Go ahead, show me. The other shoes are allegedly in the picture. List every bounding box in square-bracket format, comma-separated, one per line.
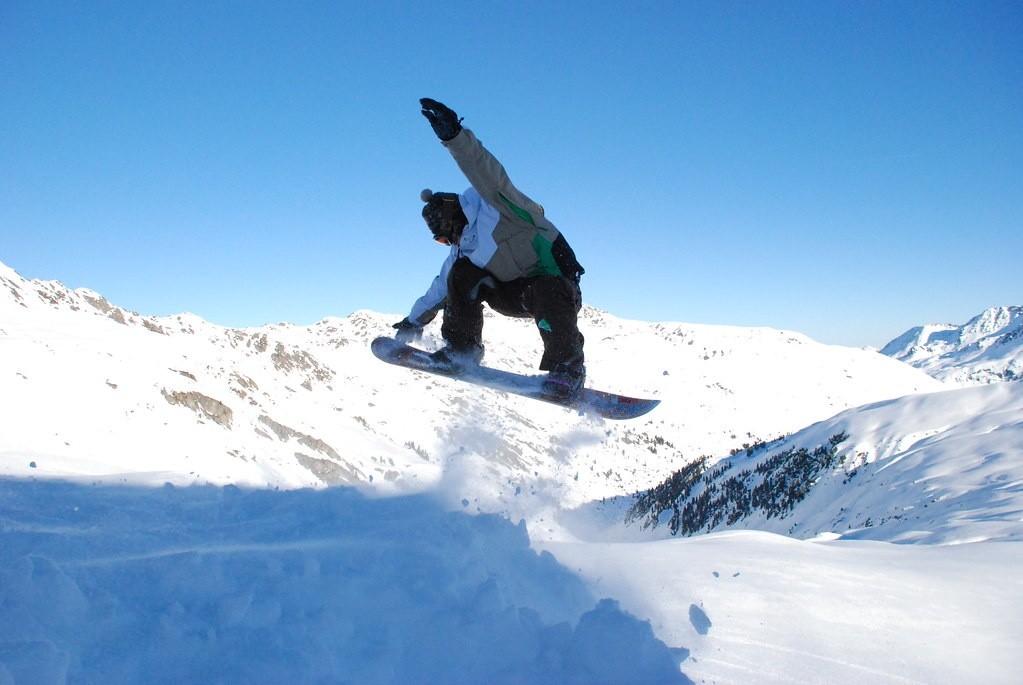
[541, 368, 586, 400]
[431, 337, 484, 373]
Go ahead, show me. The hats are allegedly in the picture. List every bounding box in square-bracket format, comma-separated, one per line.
[420, 188, 460, 237]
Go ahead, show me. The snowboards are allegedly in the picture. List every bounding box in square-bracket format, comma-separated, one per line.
[371, 336, 661, 420]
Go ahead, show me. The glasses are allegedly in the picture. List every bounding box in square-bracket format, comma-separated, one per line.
[432, 234, 454, 247]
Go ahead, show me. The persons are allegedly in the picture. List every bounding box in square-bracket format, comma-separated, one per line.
[391, 97, 585, 403]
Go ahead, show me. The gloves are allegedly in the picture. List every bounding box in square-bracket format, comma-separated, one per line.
[419, 97, 465, 141]
[392, 316, 423, 345]
